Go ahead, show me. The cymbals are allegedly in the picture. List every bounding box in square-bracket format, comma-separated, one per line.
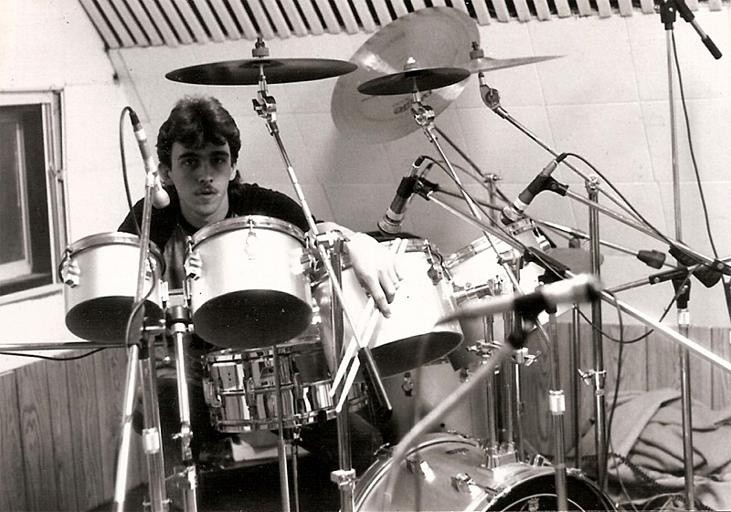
[330, 6, 481, 145]
[465, 52, 564, 75]
[359, 68, 472, 94]
[164, 56, 355, 85]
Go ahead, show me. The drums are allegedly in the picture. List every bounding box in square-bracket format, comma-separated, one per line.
[306, 238, 462, 383]
[441, 216, 584, 371]
[343, 425, 619, 512]
[199, 336, 371, 433]
[183, 214, 312, 350]
[58, 230, 166, 344]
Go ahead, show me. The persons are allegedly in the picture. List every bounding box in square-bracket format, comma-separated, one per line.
[117, 95, 404, 481]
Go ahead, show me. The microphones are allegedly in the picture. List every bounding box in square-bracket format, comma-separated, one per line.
[376, 162, 422, 238]
[405, 169, 430, 210]
[134, 122, 172, 210]
[500, 159, 559, 226]
